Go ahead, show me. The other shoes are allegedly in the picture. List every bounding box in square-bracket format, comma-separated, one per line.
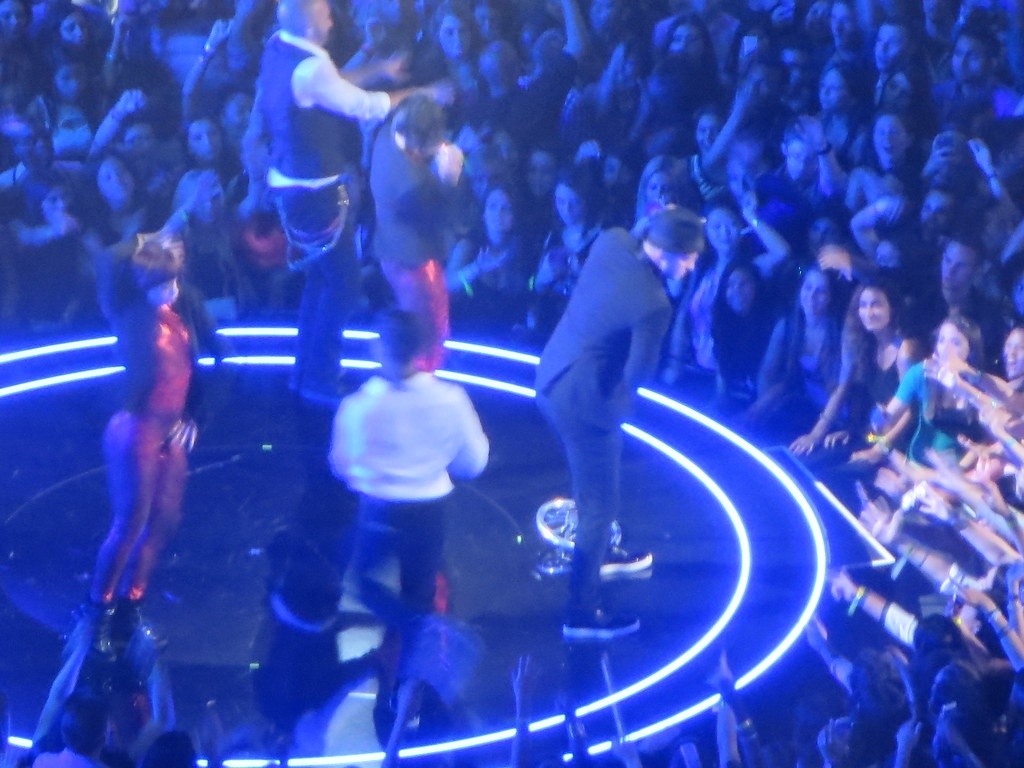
[600, 544, 654, 574]
[289, 368, 350, 411]
[564, 612, 640, 637]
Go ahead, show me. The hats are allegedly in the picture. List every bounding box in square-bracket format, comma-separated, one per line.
[648, 206, 705, 254]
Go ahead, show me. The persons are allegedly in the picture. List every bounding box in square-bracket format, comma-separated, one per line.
[91, 228, 227, 651]
[535, 209, 704, 636]
[367, 97, 449, 367]
[255, 1, 408, 407]
[272, 307, 492, 665]
[0, 0, 1024, 768]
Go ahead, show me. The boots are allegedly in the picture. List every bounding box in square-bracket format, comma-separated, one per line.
[75, 596, 119, 667]
[117, 596, 169, 651]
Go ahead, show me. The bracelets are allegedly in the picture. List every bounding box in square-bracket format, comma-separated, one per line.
[988, 174, 995, 179]
[977, 371, 982, 386]
[820, 414, 833, 423]
[868, 432, 891, 451]
[753, 219, 758, 225]
[977, 392, 981, 400]
